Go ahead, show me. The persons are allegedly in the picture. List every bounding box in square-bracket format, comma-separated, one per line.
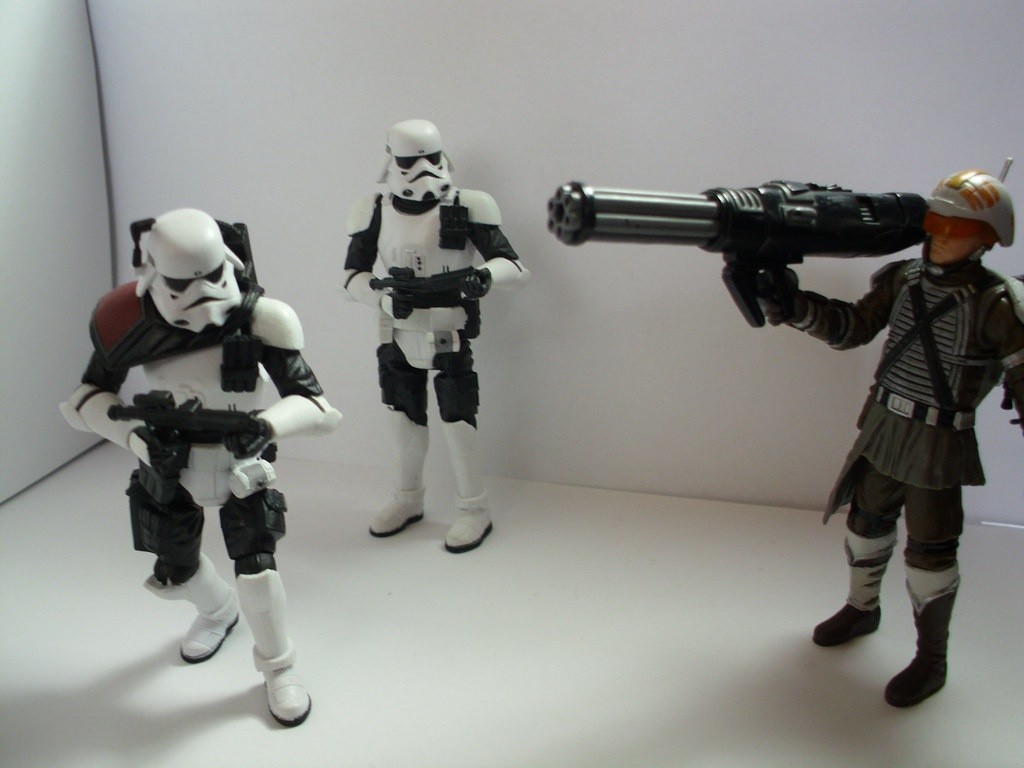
[343, 118, 531, 553]
[69, 209, 342, 727]
[757, 168, 1024, 709]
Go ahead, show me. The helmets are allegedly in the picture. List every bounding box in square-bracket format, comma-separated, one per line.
[377, 119, 455, 201]
[926, 169, 1014, 247]
[136, 208, 245, 333]
